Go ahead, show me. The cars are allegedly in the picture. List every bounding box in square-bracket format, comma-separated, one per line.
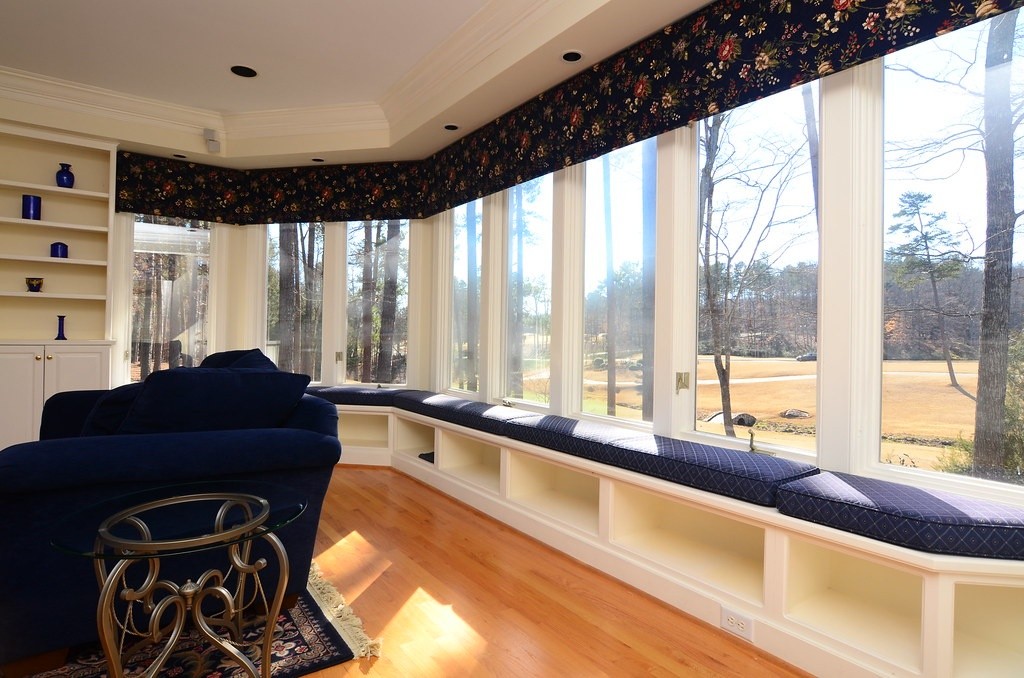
[796, 353, 817, 362]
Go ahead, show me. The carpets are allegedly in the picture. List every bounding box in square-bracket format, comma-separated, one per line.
[30, 561, 379, 678]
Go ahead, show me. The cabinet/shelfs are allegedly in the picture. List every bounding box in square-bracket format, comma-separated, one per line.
[0, 124, 119, 454]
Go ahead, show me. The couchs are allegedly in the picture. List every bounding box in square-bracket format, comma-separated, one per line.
[0, 395, 341, 668]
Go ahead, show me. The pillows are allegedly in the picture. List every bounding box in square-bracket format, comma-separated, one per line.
[77, 349, 310, 437]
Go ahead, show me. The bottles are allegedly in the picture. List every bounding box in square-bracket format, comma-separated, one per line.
[25, 278, 43, 292]
[54, 315, 67, 340]
[55, 162, 74, 188]
[50, 242, 68, 258]
[21, 195, 42, 220]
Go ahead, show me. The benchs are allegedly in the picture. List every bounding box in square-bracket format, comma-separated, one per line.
[303, 383, 1024, 678]
